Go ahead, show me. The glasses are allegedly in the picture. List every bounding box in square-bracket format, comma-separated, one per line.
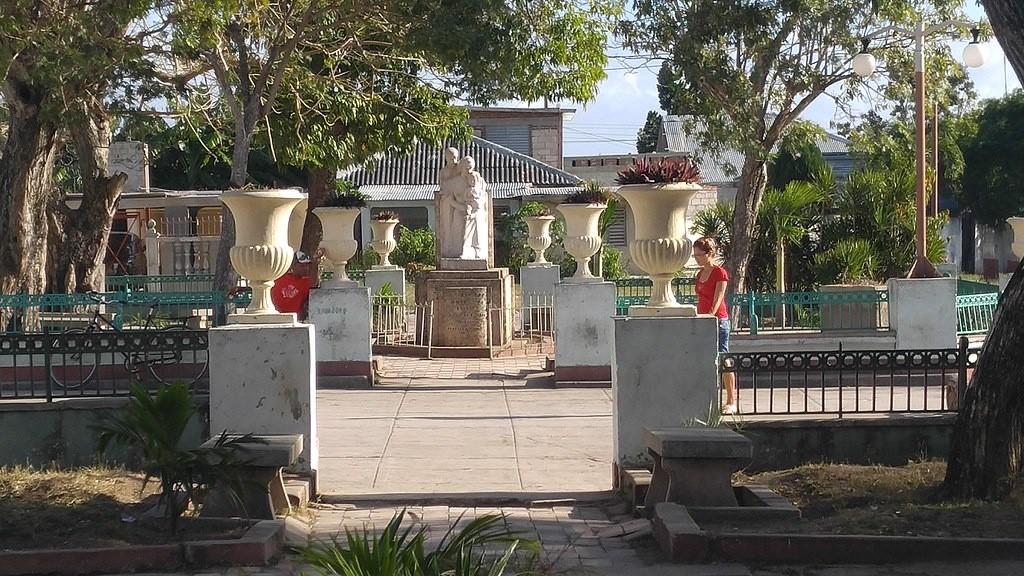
[692, 251, 709, 258]
[292, 255, 310, 266]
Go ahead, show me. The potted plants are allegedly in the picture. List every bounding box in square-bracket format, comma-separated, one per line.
[522, 209, 556, 266]
[311, 188, 367, 289]
[218, 184, 305, 324]
[368, 210, 400, 270]
[613, 154, 703, 317]
[556, 179, 612, 282]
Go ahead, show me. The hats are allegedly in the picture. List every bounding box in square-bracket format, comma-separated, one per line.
[293, 250, 312, 265]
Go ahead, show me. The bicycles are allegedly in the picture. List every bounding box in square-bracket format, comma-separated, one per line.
[47, 290, 210, 389]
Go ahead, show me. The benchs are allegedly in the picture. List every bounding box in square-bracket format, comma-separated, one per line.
[645, 425, 753, 514]
[199, 433, 304, 520]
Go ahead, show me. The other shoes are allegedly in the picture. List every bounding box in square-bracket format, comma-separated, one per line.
[723, 404, 736, 413]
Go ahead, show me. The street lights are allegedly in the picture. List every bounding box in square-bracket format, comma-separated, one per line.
[851, 11, 987, 278]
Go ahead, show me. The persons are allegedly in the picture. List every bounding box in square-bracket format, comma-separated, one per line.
[693, 237, 736, 413]
[270, 249, 323, 320]
[440, 148, 487, 258]
